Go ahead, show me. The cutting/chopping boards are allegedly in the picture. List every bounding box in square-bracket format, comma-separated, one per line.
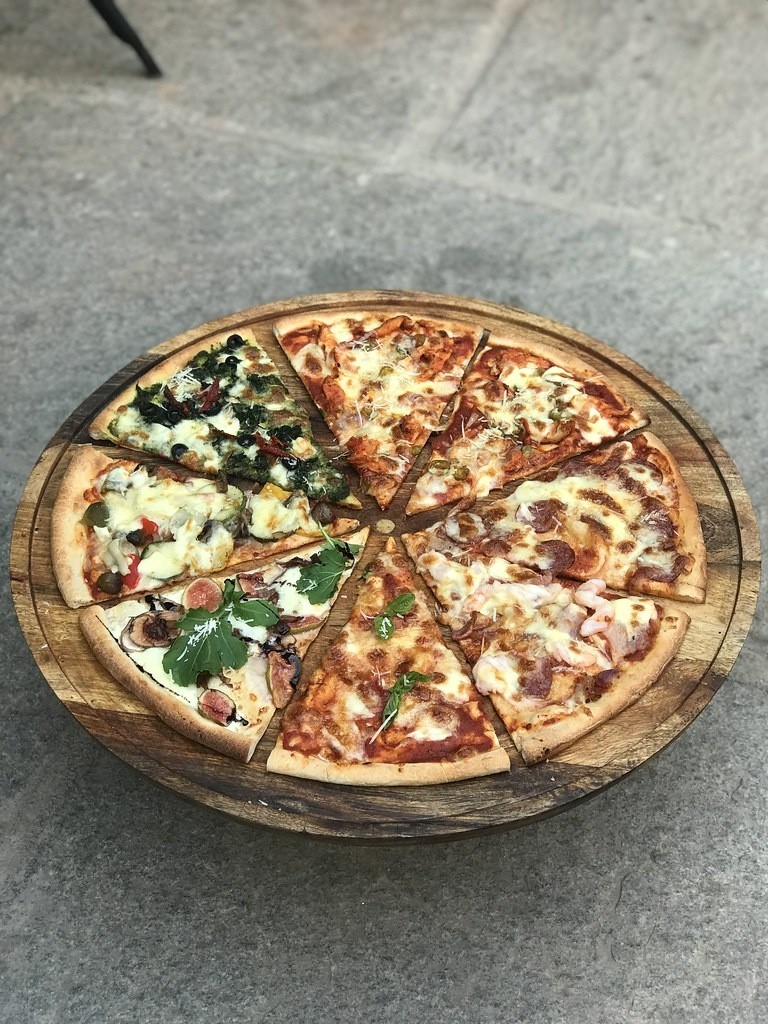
[10, 288, 760, 839]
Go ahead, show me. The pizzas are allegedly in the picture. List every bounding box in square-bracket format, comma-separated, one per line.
[50, 310, 705, 786]
